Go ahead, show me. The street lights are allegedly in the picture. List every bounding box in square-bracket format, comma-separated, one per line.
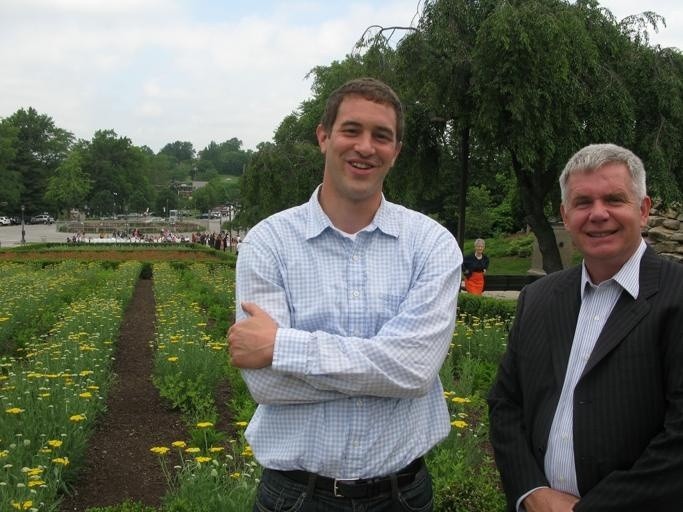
[21, 205, 26, 243]
[225, 202, 234, 252]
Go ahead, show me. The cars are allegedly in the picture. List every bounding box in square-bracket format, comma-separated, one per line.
[196, 213, 222, 220]
[0, 216, 10, 225]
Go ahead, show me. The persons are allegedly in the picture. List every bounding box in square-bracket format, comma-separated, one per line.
[65, 221, 242, 255]
[225, 76, 464, 512]
[485, 143, 683, 512]
[462, 239, 490, 296]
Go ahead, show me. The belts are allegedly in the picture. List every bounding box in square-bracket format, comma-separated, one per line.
[285, 459, 424, 497]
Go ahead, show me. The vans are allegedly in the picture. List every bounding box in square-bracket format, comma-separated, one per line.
[30, 214, 50, 224]
